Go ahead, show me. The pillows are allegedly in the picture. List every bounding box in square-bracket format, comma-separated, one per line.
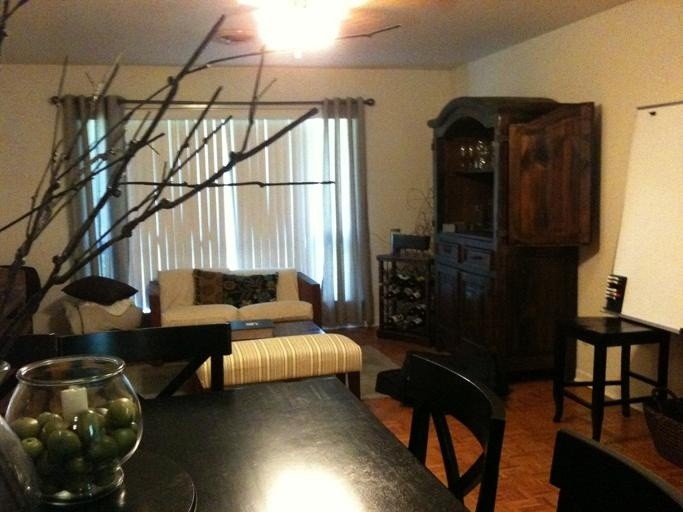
[188, 267, 224, 304]
[220, 271, 279, 307]
[60, 274, 138, 307]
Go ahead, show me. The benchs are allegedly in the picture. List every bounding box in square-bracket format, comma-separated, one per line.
[194, 331, 363, 402]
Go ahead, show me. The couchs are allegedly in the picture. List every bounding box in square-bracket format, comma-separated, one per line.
[145, 264, 322, 327]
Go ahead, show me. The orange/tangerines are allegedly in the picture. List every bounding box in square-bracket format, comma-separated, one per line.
[12, 397, 138, 494]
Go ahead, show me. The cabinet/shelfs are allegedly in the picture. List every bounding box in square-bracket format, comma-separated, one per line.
[423, 88, 604, 251]
[372, 248, 435, 348]
[431, 231, 577, 390]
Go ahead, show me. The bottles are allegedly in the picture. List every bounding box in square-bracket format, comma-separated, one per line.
[5, 356, 141, 508]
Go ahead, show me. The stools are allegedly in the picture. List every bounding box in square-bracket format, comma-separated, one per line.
[29, 282, 141, 334]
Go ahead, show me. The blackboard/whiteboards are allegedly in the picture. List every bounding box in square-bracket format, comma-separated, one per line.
[602, 100, 683, 336]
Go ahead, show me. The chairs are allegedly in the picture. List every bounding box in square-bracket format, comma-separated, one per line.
[397, 348, 507, 511]
[0, 332, 49, 400]
[0, 262, 42, 337]
[45, 319, 232, 405]
[543, 428, 680, 511]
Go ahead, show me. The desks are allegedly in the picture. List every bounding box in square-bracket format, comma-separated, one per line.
[0, 373, 476, 510]
[547, 309, 672, 445]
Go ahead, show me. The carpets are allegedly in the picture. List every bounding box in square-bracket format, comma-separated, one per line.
[332, 342, 399, 403]
